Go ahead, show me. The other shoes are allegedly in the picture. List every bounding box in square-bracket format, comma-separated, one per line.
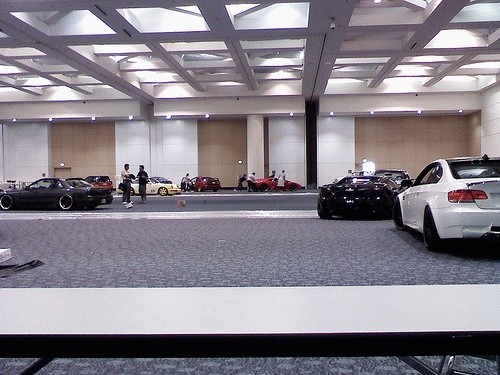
[140, 201, 144, 203]
[143, 200, 146, 204]
[122, 201, 128, 204]
[129, 201, 134, 204]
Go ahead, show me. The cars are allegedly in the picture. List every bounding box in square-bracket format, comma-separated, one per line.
[0, 176, 103, 211]
[317, 168, 416, 220]
[250, 177, 302, 193]
[116, 176, 181, 196]
[391, 153, 500, 253]
[85, 175, 113, 195]
[185, 176, 222, 192]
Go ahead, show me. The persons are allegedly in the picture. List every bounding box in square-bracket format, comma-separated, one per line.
[233, 172, 256, 192]
[136, 164, 149, 204]
[277, 170, 286, 192]
[121, 164, 135, 205]
[181, 173, 191, 192]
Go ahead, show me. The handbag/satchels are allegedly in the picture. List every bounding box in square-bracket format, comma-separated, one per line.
[118, 183, 127, 193]
